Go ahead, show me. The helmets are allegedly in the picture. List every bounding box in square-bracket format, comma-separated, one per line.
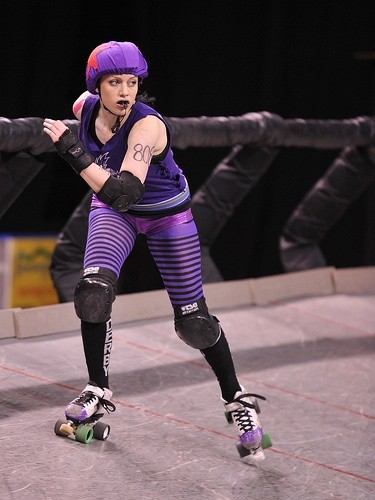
[86, 41, 148, 95]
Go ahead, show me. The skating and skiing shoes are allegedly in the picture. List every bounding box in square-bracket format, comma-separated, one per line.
[221, 384, 272, 461]
[55, 381, 113, 444]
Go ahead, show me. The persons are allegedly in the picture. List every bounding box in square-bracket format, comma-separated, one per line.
[42, 41, 274, 459]
